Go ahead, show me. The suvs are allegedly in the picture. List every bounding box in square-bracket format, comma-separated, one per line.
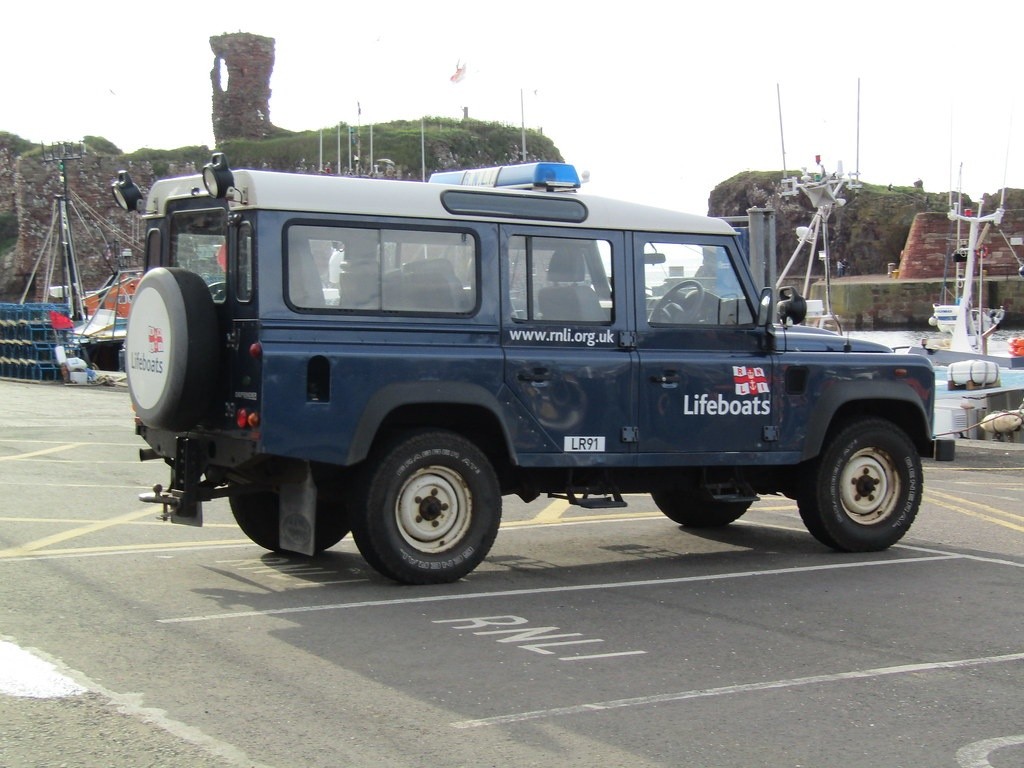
[112, 152, 936, 588]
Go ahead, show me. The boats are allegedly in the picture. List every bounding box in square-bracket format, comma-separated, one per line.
[767, 154, 1024, 444]
[18, 138, 145, 372]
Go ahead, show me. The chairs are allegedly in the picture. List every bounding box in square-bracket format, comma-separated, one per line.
[535, 245, 607, 323]
[336, 240, 392, 309]
[388, 257, 469, 313]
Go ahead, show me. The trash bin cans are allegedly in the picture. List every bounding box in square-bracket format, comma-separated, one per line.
[888, 263, 895, 277]
